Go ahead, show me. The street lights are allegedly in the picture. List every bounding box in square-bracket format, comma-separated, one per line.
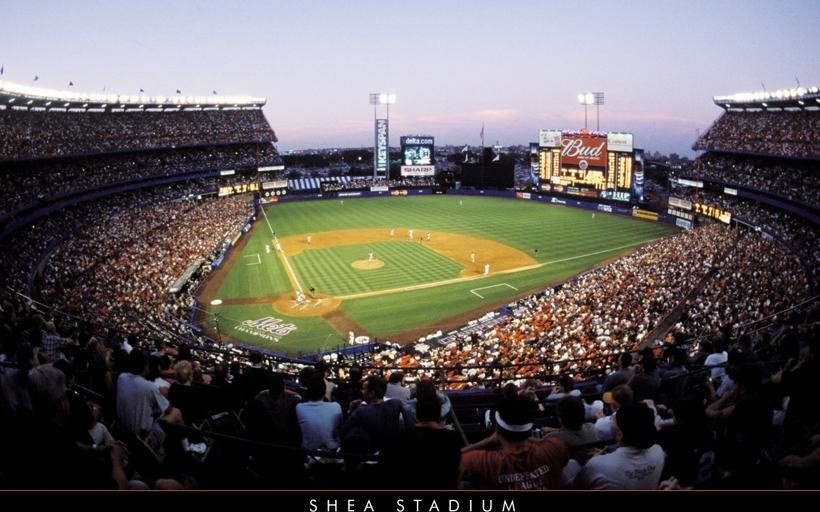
[576, 91, 594, 128]
[380, 94, 393, 179]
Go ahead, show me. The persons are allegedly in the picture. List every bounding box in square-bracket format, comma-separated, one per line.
[600, 106, 818, 492]
[419, 144, 431, 164]
[340, 199, 345, 206]
[390, 228, 395, 236]
[320, 174, 440, 192]
[409, 229, 414, 239]
[427, 232, 431, 241]
[406, 146, 418, 165]
[280, 265, 599, 489]
[460, 200, 462, 207]
[307, 235, 312, 243]
[368, 250, 374, 260]
[471, 253, 476, 262]
[0, 94, 280, 490]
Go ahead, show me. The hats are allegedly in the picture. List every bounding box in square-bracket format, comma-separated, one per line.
[602, 384, 633, 408]
